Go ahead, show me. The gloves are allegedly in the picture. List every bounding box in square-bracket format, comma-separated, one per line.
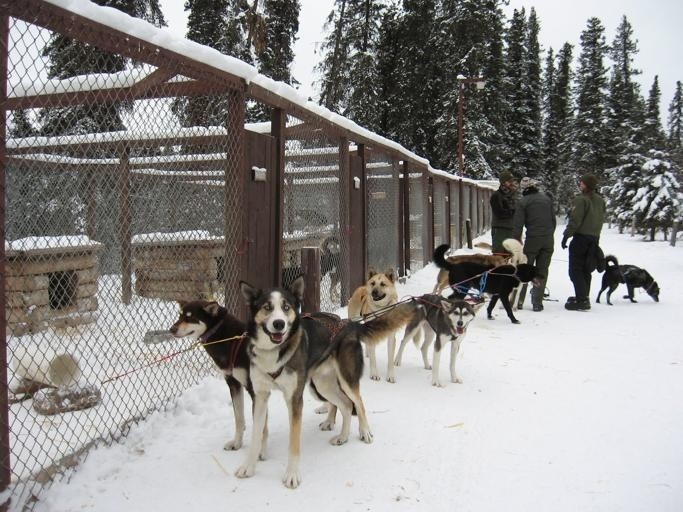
[561, 237, 567, 249]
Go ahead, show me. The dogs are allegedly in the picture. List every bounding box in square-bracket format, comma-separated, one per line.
[596, 255, 660, 305]
[234, 274, 417, 488]
[348, 237, 547, 388]
[169, 297, 338, 451]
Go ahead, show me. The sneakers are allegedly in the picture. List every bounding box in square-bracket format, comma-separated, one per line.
[565, 296, 590, 310]
[533, 302, 543, 311]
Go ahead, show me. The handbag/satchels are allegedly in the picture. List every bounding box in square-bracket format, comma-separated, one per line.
[584, 241, 605, 273]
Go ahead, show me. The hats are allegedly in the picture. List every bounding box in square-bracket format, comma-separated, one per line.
[498, 172, 519, 185]
[520, 177, 541, 194]
[575, 172, 598, 190]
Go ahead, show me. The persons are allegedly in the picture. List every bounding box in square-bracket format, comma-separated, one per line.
[514, 178, 557, 313]
[561, 173, 606, 312]
[488, 170, 522, 263]
